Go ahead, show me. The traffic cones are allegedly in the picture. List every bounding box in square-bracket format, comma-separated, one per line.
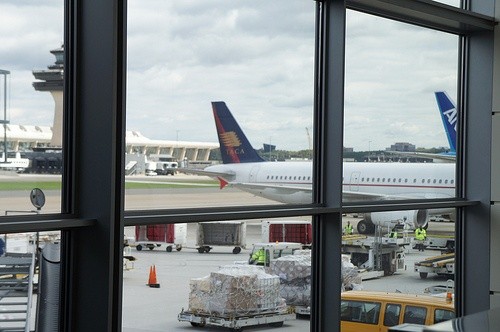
[146, 264, 160, 288]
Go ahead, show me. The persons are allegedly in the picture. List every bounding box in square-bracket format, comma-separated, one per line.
[250, 246, 267, 267]
[344, 221, 354, 235]
[387, 228, 397, 239]
[415, 225, 427, 252]
[124, 239, 129, 248]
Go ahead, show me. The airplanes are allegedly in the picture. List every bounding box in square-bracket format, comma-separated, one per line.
[163, 89, 457, 235]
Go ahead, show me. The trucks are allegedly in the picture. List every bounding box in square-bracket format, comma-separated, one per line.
[414, 250, 455, 280]
[247, 240, 304, 269]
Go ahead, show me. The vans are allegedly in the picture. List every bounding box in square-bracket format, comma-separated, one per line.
[341, 288, 455, 332]
